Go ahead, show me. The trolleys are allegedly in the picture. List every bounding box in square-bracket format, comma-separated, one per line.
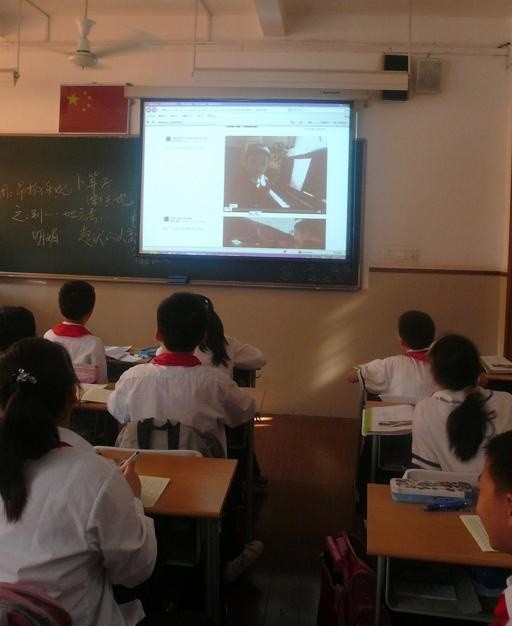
[36, 0, 149, 67]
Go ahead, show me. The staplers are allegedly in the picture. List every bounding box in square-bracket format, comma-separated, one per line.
[167, 274, 189, 285]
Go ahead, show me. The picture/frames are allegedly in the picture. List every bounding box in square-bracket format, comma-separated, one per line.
[237, 367, 261, 388]
[72, 383, 118, 445]
[366, 400, 415, 482]
[94, 448, 239, 617]
[367, 483, 512, 626]
[238, 387, 265, 539]
[105, 356, 132, 382]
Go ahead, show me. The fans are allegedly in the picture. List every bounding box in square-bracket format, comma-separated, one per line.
[0, 134, 367, 291]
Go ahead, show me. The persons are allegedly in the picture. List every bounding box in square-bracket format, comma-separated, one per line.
[476, 431, 512, 626]
[155, 295, 266, 384]
[412, 334, 510, 473]
[42, 280, 108, 386]
[226, 143, 272, 207]
[1, 337, 157, 626]
[348, 310, 442, 404]
[0, 306, 36, 343]
[106, 291, 257, 459]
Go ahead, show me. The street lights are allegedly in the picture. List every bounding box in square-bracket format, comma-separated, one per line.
[316, 531, 388, 626]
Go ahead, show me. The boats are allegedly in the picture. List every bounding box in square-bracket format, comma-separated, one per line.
[382, 56, 408, 101]
[416, 57, 444, 94]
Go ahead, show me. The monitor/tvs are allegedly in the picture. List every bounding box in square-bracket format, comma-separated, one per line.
[67, 381, 113, 403]
[362, 405, 416, 437]
[478, 351, 510, 376]
[103, 340, 136, 366]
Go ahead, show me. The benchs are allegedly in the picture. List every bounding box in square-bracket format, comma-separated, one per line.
[60, 85, 127, 133]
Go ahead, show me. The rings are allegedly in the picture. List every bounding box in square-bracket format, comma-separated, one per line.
[120, 450, 139, 471]
[424, 500, 467, 512]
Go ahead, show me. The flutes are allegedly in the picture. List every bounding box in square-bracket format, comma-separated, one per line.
[390, 478, 473, 506]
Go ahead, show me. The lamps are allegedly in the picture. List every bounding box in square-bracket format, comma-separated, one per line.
[94, 446, 204, 567]
[72, 364, 103, 440]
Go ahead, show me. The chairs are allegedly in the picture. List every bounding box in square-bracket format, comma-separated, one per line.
[224, 541, 264, 582]
